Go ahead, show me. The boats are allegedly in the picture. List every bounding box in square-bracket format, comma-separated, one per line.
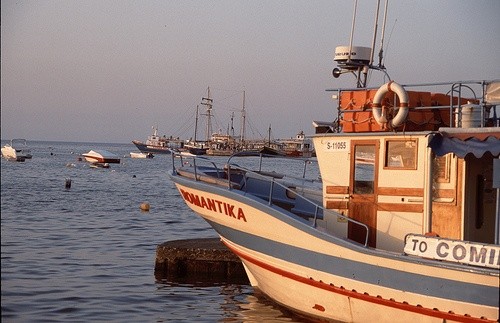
[132, 125, 188, 154]
[129, 152, 153, 159]
[205, 123, 316, 158]
[168, 148, 201, 158]
[1, 138, 32, 161]
[81, 149, 120, 164]
[166, 0, 499, 322]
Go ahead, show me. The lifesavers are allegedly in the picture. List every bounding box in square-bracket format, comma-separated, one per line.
[372, 80, 409, 127]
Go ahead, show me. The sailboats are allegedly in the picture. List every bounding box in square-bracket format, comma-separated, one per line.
[182, 86, 286, 157]
